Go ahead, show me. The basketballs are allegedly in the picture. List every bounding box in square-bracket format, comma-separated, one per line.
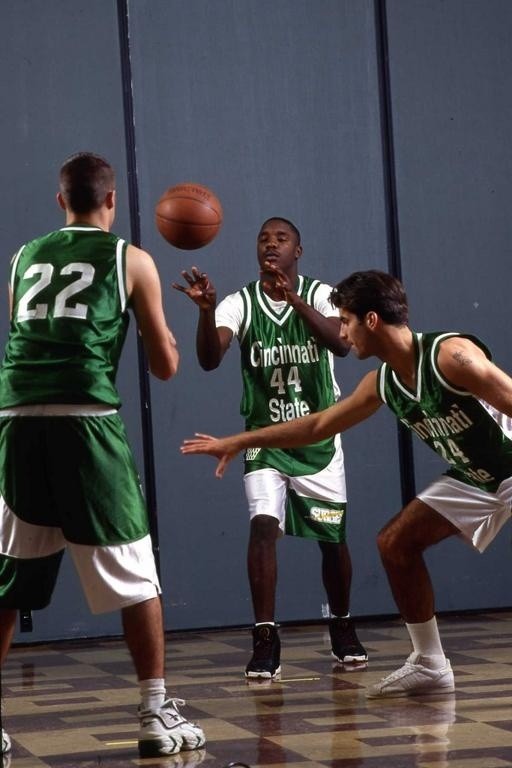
[155, 182, 224, 251]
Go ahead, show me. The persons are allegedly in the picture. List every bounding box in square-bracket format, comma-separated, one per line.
[250, 669, 366, 766]
[170, 217, 369, 680]
[137, 747, 209, 768]
[367, 698, 457, 767]
[0, 150, 208, 756]
[178, 267, 510, 701]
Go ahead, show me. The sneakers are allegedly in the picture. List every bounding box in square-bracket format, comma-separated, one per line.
[246, 624, 282, 679]
[365, 650, 455, 698]
[1, 728, 12, 752]
[326, 611, 368, 663]
[136, 696, 206, 757]
